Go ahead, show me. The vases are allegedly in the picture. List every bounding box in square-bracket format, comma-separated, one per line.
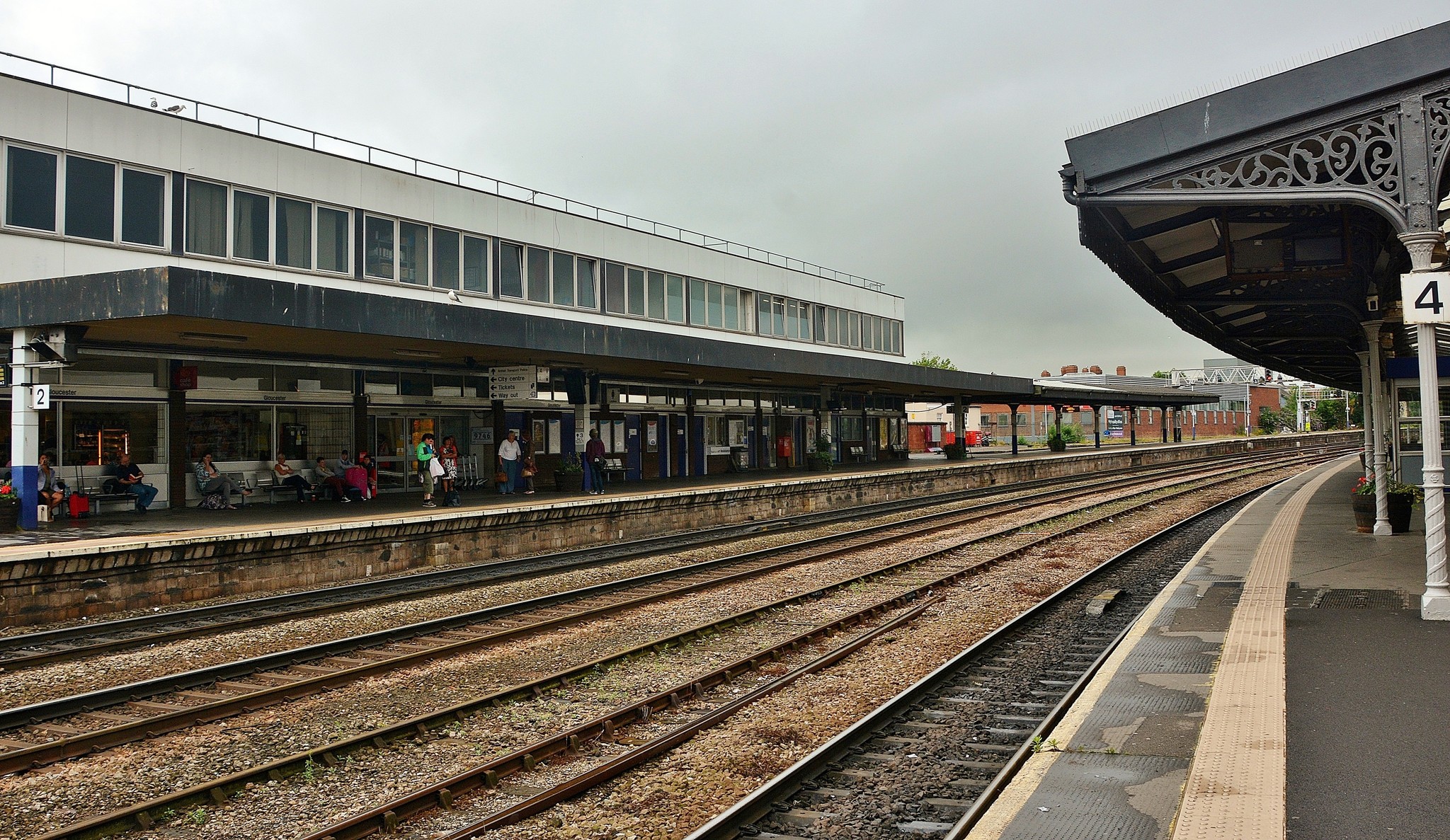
[1352, 493, 1414, 534]
[553, 472, 584, 492]
[0, 498, 22, 535]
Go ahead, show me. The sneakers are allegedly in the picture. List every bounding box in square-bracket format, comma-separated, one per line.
[361, 496, 367, 501]
[589, 490, 598, 494]
[600, 490, 604, 494]
[422, 501, 436, 507]
[340, 497, 351, 503]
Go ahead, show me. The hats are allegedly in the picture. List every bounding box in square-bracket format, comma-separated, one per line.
[341, 450, 348, 455]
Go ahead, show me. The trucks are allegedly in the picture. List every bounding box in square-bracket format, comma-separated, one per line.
[946, 430, 982, 448]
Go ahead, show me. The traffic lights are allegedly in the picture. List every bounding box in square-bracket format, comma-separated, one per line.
[1311, 399, 1317, 409]
[1264, 368, 1273, 380]
[1337, 389, 1342, 398]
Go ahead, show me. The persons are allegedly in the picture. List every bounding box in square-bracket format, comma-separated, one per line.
[117, 454, 159, 516]
[37, 454, 64, 523]
[314, 457, 351, 504]
[438, 436, 457, 492]
[416, 433, 439, 507]
[274, 453, 320, 505]
[521, 430, 536, 494]
[585, 429, 605, 494]
[195, 452, 251, 511]
[360, 455, 376, 499]
[450, 436, 459, 494]
[334, 451, 364, 500]
[498, 431, 521, 495]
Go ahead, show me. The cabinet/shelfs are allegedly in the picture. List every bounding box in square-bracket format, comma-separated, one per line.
[368, 238, 416, 284]
[187, 427, 249, 460]
[74, 425, 130, 464]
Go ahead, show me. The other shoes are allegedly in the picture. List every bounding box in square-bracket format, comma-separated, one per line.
[226, 505, 237, 509]
[50, 509, 53, 519]
[511, 491, 515, 494]
[242, 491, 250, 499]
[502, 492, 505, 494]
[523, 490, 535, 494]
[135, 503, 147, 512]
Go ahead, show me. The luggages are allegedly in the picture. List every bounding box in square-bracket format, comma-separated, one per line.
[70, 462, 90, 519]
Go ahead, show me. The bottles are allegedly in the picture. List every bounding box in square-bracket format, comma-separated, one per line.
[45, 460, 49, 463]
[64, 484, 71, 497]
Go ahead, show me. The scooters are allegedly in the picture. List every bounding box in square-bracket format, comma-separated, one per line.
[981, 432, 991, 447]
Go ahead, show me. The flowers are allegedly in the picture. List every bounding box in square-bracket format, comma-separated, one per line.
[554, 451, 584, 473]
[0, 478, 18, 501]
[1350, 461, 1424, 511]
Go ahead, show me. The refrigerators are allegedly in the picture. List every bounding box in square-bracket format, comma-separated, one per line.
[73, 412, 129, 465]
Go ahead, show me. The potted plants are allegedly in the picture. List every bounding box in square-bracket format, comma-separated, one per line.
[1047, 439, 1066, 451]
[943, 443, 963, 459]
[807, 430, 834, 471]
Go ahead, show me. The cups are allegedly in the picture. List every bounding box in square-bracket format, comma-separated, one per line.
[311, 495, 316, 501]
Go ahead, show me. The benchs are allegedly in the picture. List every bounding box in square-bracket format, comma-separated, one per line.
[850, 447, 873, 463]
[195, 472, 253, 504]
[256, 468, 336, 504]
[892, 444, 911, 461]
[602, 459, 634, 482]
[77, 477, 153, 515]
[50, 479, 70, 518]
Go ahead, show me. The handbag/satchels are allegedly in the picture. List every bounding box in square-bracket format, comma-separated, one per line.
[593, 454, 606, 470]
[492, 464, 508, 483]
[202, 494, 228, 510]
[56, 482, 68, 497]
[520, 467, 534, 478]
[442, 481, 463, 507]
[103, 478, 132, 494]
[429, 458, 445, 478]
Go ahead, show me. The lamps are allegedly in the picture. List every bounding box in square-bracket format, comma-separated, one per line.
[544, 360, 584, 368]
[749, 376, 772, 382]
[393, 349, 442, 358]
[660, 369, 691, 376]
[178, 332, 249, 343]
[818, 382, 973, 397]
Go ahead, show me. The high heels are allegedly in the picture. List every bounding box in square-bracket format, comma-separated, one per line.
[298, 498, 304, 504]
[309, 485, 318, 492]
